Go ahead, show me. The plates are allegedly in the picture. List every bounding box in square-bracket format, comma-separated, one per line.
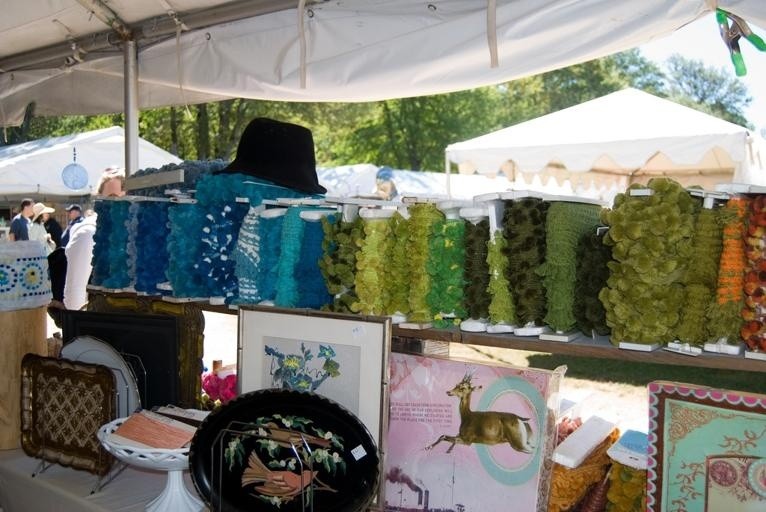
[56, 336, 141, 419]
[20, 353, 113, 477]
[189, 387, 382, 510]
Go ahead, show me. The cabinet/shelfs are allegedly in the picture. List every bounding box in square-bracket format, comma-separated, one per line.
[0, 304, 46, 452]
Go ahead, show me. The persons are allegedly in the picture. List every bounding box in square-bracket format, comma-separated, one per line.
[8, 167, 124, 328]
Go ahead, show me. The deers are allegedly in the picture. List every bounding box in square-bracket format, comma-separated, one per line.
[424, 363, 536, 455]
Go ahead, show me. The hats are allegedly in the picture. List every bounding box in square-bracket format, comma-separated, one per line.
[212, 116, 329, 196]
[64, 203, 82, 213]
[31, 203, 56, 222]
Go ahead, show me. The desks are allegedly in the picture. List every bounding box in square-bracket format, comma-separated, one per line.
[0, 417, 213, 512]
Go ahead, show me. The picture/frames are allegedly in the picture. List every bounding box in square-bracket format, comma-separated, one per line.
[640, 377, 766, 512]
[233, 304, 393, 512]
